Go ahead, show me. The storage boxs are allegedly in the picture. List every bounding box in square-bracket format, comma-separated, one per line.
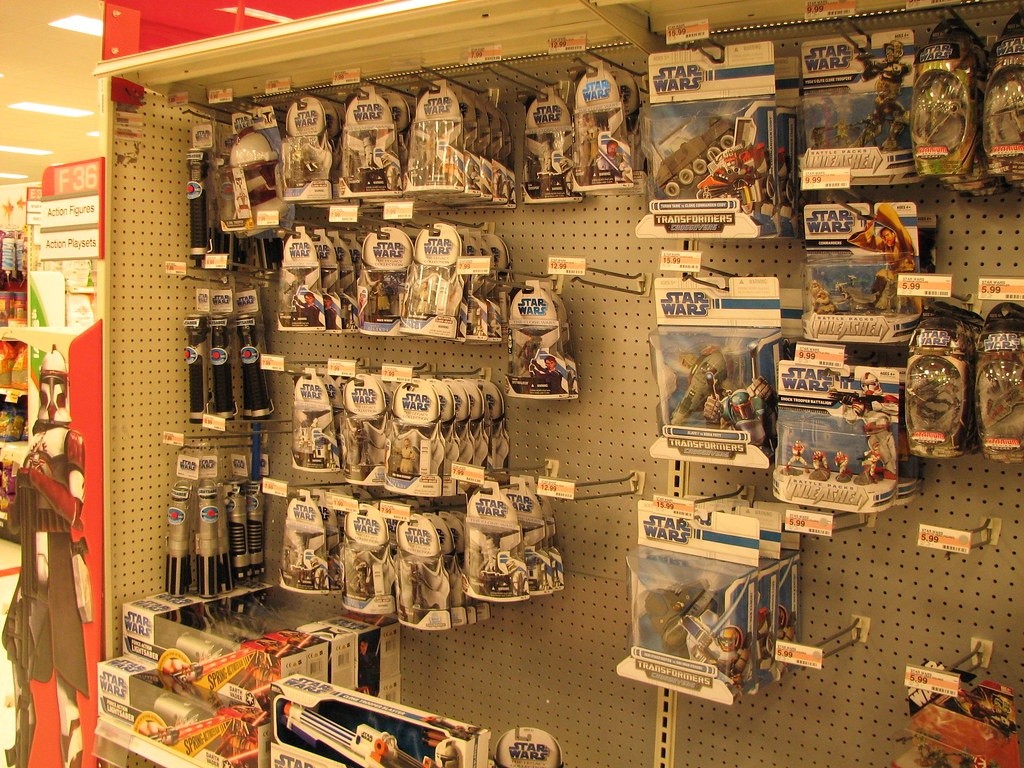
[97, 581, 491, 768]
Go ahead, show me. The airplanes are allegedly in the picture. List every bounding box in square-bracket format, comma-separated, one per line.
[668, 347, 748, 430]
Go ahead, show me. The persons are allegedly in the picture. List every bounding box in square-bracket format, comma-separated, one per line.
[855, 40, 911, 149]
[4, 346, 93, 767]
[785, 440, 882, 480]
[854, 372, 895, 479]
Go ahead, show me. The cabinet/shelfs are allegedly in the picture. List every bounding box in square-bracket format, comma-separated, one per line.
[0, 156, 104, 555]
[94, 2, 1023, 768]
